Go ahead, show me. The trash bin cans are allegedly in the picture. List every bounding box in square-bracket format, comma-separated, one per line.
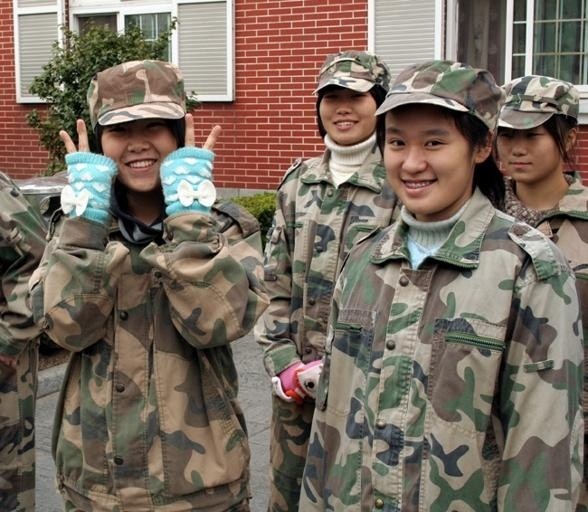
[17, 176, 69, 226]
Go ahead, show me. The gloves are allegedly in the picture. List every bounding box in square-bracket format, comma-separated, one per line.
[271, 357, 324, 405]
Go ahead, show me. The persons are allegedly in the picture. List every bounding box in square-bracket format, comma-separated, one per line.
[0, 172, 48, 512]
[489, 75, 588, 512]
[253, 53, 402, 512]
[296, 60, 585, 512]
[25, 59, 269, 512]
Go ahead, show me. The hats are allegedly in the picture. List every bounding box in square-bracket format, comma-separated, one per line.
[312, 50, 390, 94]
[86, 59, 187, 133]
[497, 76, 581, 130]
[374, 61, 506, 131]
